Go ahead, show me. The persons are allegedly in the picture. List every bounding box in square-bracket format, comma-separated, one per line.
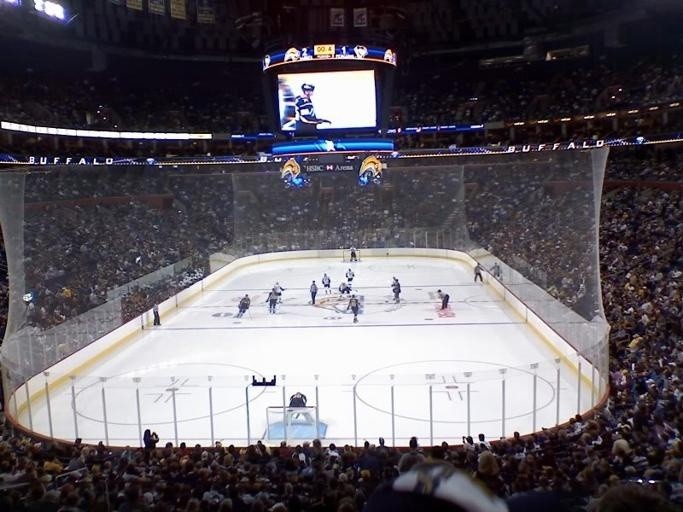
[0, 44, 683, 512]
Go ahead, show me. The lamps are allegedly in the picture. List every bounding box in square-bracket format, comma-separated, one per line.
[29, 0, 70, 25]
[0, 0, 24, 9]
